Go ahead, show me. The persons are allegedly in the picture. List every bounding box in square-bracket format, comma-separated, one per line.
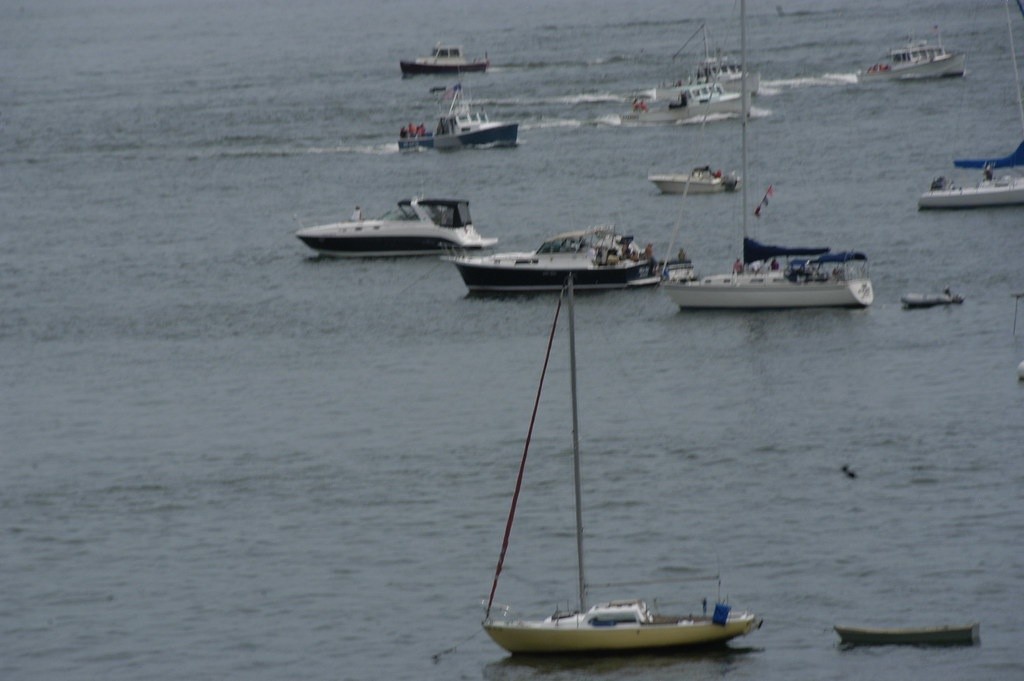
[769, 259, 779, 270]
[983, 164, 993, 183]
[644, 242, 656, 276]
[400, 122, 426, 137]
[560, 236, 641, 265]
[678, 248, 685, 260]
[351, 206, 362, 222]
[733, 258, 743, 275]
[432, 205, 453, 225]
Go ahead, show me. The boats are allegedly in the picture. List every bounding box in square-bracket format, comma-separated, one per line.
[398, 65, 519, 152]
[901, 284, 966, 306]
[828, 621, 984, 647]
[918, 1, 1024, 206]
[618, 24, 751, 124]
[295, 169, 499, 258]
[400, 42, 489, 76]
[649, 161, 741, 194]
[440, 222, 697, 294]
[698, 22, 760, 94]
[855, 21, 967, 85]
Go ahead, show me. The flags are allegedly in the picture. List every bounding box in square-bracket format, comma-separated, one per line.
[755, 187, 773, 217]
[441, 83, 462, 100]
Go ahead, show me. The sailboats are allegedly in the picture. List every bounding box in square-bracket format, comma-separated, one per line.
[661, 0, 875, 309]
[478, 268, 766, 652]
